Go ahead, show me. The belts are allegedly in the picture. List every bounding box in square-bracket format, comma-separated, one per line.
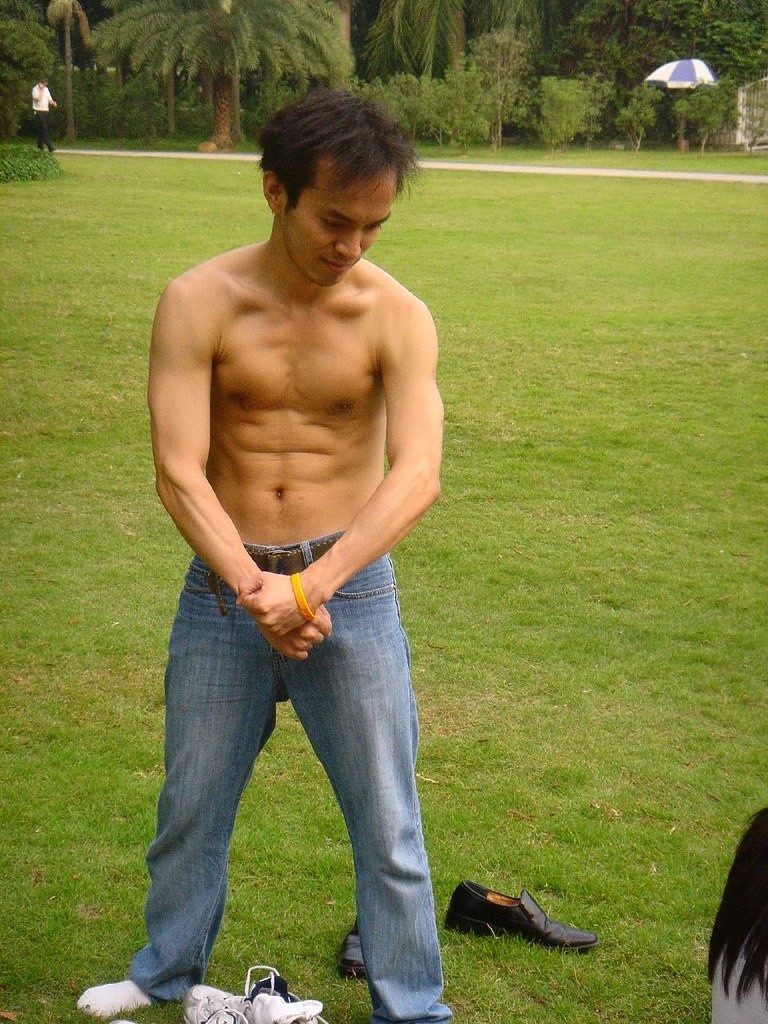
[244, 539, 339, 576]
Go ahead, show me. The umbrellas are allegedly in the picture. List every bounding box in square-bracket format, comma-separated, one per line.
[643, 58, 720, 90]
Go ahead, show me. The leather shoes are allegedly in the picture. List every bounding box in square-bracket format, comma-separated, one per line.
[339, 917, 367, 974]
[443, 881, 599, 952]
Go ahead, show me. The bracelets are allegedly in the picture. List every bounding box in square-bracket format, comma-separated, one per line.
[291, 572, 317, 622]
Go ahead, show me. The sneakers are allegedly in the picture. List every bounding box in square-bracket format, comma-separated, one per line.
[182, 965, 328, 1024]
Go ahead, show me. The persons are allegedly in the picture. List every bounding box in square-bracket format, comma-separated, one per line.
[31, 78, 57, 153]
[708, 808, 768, 1024]
[76, 90, 445, 1024]
